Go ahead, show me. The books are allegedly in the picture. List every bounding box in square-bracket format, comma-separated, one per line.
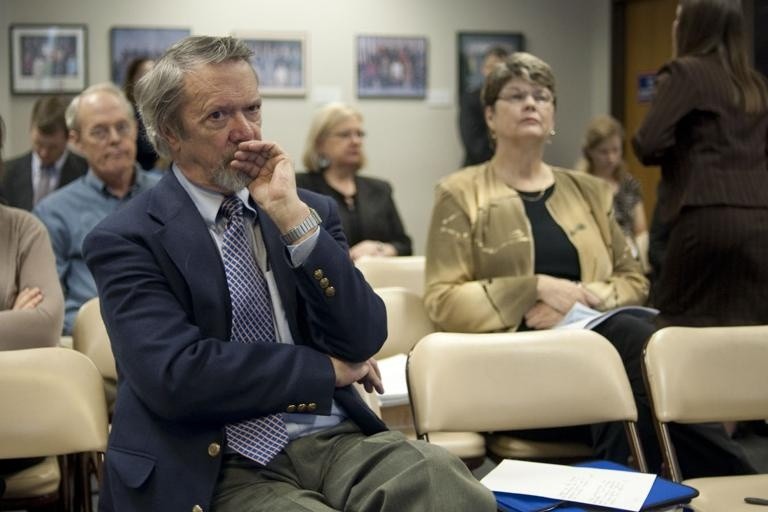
[550, 301, 662, 342]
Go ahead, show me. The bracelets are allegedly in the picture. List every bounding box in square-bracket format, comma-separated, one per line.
[280, 207, 322, 245]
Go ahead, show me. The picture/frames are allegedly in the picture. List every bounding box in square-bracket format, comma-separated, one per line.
[8, 22, 88, 96]
[108, 26, 192, 88]
[457, 30, 527, 106]
[226, 31, 309, 97]
[357, 33, 427, 100]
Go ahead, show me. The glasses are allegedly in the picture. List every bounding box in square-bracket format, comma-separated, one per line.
[497, 89, 554, 104]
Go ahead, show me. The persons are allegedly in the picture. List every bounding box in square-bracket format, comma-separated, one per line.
[630, 1, 767, 329]
[575, 113, 649, 262]
[292, 100, 414, 262]
[419, 50, 759, 480]
[80, 33, 495, 512]
[123, 56, 162, 170]
[0, 89, 89, 212]
[27, 81, 163, 337]
[0, 202, 65, 509]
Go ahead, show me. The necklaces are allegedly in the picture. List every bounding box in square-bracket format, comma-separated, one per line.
[506, 175, 548, 204]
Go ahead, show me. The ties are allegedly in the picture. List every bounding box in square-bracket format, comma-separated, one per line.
[34, 163, 49, 207]
[220, 193, 289, 467]
